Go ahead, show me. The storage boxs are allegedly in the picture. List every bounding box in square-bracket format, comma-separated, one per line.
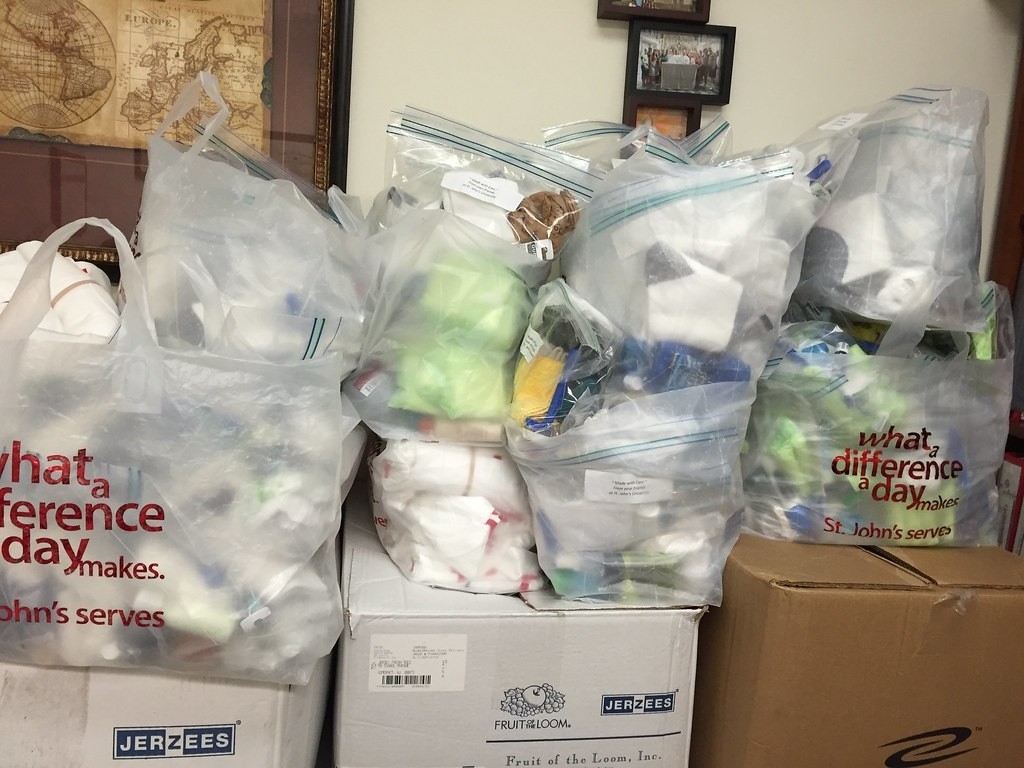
[994, 451, 1023, 556]
[333, 486, 708, 768]
[688, 537, 1024, 768]
[0, 652, 329, 768]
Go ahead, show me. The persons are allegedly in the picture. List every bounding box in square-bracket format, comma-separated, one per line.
[640, 45, 718, 93]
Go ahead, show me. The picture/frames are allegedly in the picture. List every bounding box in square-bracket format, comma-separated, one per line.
[597, 0, 711, 24]
[624, 20, 737, 106]
[0, 0, 356, 285]
[620, 94, 703, 159]
